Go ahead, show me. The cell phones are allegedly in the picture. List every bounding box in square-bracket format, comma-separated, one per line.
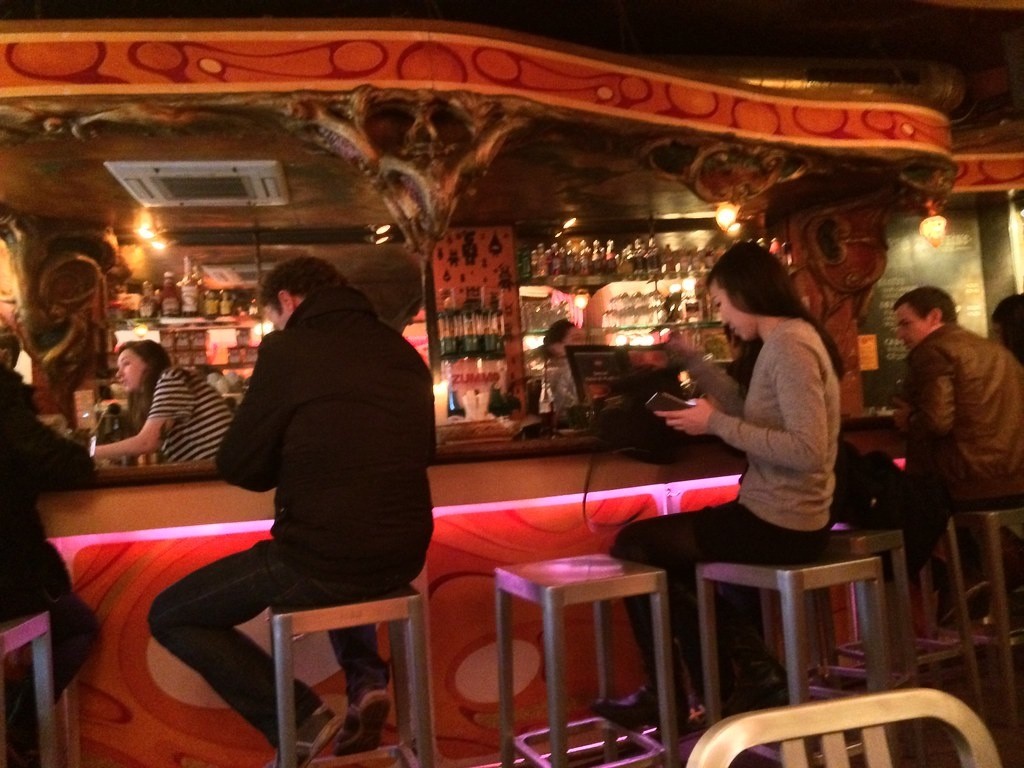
[646, 391, 692, 411]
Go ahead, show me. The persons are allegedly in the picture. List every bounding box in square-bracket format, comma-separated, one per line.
[871, 286, 1024, 628]
[148, 256, 436, 768]
[93, 340, 233, 462]
[543, 320, 585, 415]
[0, 317, 102, 768]
[592, 240, 844, 737]
[991, 294, 1024, 366]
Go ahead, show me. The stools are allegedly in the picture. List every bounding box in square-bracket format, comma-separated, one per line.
[921, 503, 1024, 731]
[697, 553, 899, 768]
[267, 584, 434, 768]
[806, 529, 925, 765]
[0, 609, 59, 768]
[495, 553, 682, 768]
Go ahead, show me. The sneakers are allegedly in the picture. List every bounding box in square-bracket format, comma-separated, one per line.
[267, 702, 343, 768]
[332, 682, 390, 757]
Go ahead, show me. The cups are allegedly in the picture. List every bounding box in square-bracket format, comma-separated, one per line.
[679, 252, 701, 272]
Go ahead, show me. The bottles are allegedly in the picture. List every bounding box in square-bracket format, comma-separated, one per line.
[751, 237, 794, 266]
[437, 307, 508, 354]
[518, 233, 677, 275]
[136, 254, 235, 319]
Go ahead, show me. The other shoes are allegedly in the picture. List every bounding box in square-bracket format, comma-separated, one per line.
[1006, 590, 1024, 636]
[588, 685, 690, 731]
[720, 659, 789, 721]
[937, 580, 991, 627]
